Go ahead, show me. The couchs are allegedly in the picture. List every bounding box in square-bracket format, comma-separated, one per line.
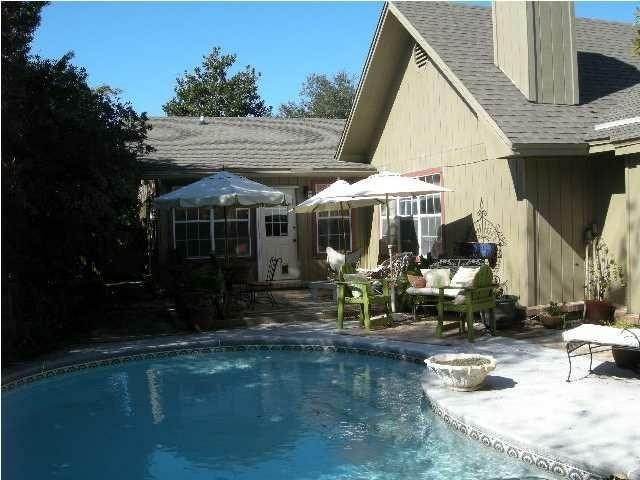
[407, 265, 481, 310]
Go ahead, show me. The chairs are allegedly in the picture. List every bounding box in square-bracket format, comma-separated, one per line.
[248, 256, 282, 307]
[336, 264, 392, 330]
[436, 264, 496, 342]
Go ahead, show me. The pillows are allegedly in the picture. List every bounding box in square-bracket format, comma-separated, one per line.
[420, 269, 450, 288]
[343, 274, 375, 298]
[450, 266, 480, 287]
[452, 278, 474, 304]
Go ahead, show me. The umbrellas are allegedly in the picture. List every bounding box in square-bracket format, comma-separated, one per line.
[152, 164, 292, 289]
[287, 177, 392, 264]
[306, 227, 307, 228]
[325, 168, 455, 313]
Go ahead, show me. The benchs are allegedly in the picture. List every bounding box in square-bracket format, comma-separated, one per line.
[562, 324, 640, 382]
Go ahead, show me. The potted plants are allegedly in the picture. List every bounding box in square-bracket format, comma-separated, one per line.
[582, 236, 627, 322]
